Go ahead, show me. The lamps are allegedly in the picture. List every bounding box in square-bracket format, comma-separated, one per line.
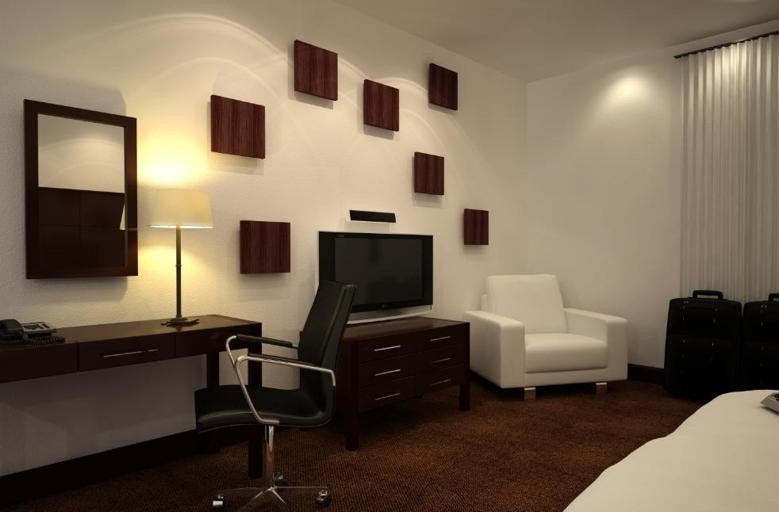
[150, 188, 211, 325]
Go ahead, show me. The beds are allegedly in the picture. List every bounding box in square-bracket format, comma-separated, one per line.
[563, 389, 779, 512]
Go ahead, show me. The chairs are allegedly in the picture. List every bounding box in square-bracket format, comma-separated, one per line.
[466, 274, 627, 401]
[194, 280, 357, 507]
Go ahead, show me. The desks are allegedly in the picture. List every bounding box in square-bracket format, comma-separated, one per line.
[0, 314, 268, 480]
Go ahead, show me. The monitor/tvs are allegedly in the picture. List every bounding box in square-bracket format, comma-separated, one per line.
[315, 230, 435, 326]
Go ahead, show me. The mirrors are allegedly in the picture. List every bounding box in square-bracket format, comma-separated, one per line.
[23, 98, 138, 280]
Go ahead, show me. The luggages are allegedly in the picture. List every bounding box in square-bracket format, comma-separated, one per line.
[662, 289, 742, 397]
[743, 293, 779, 390]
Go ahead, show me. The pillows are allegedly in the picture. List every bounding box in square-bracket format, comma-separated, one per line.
[486, 274, 568, 333]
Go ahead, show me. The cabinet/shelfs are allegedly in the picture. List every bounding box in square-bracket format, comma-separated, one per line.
[299, 322, 470, 450]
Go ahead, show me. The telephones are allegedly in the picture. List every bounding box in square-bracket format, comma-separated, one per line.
[0, 319, 55, 339]
